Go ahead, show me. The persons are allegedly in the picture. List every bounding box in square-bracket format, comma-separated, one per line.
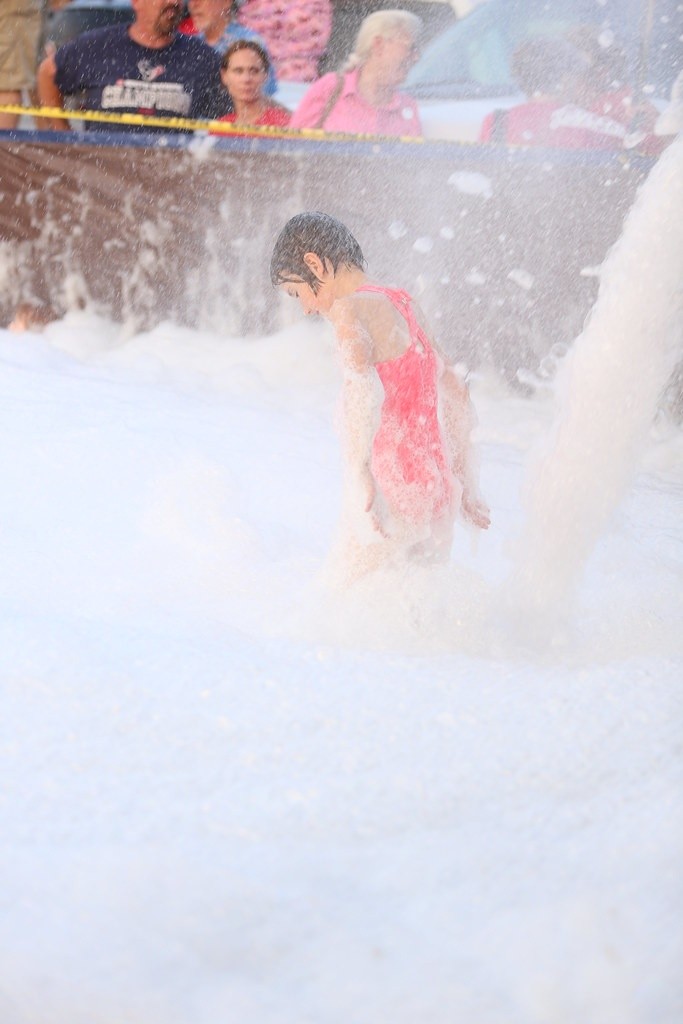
[38, 0, 293, 134]
[205, 39, 291, 138]
[567, 24, 660, 147]
[271, 210, 492, 564]
[0, 0, 50, 131]
[285, 8, 423, 137]
[188, 0, 279, 135]
[480, 33, 633, 150]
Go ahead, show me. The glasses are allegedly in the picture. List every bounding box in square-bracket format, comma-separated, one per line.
[388, 38, 417, 54]
